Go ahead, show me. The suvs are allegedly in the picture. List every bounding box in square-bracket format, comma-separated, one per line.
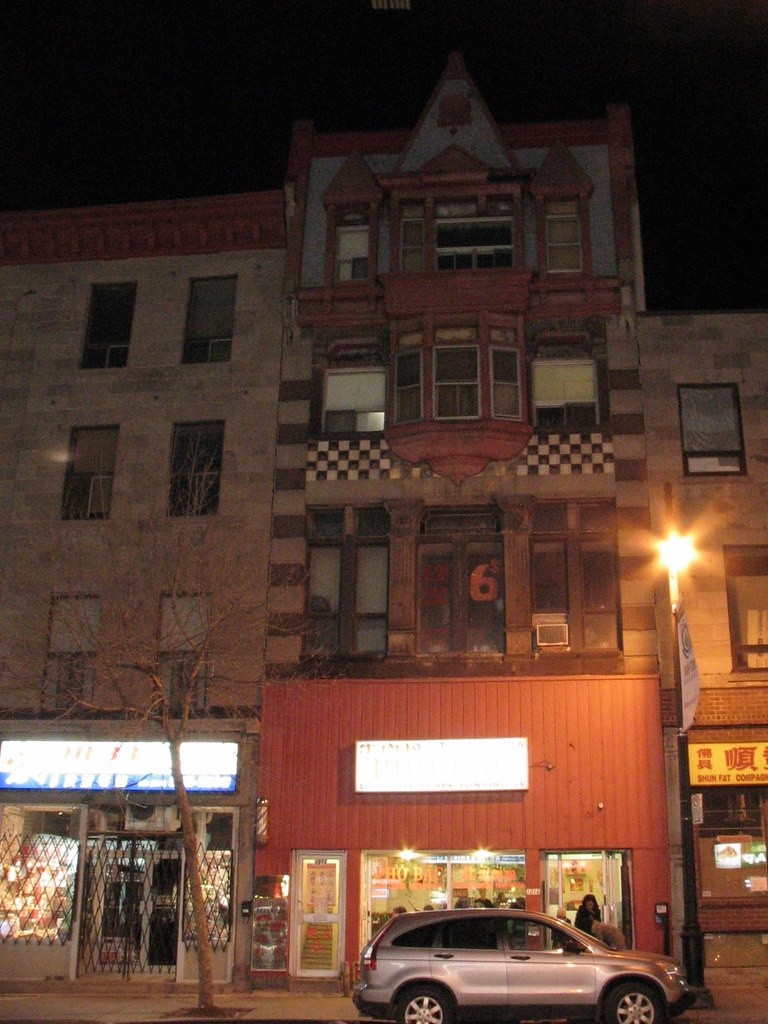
[353, 907, 697, 1024]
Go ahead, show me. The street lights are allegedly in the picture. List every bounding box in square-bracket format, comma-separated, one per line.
[655, 528, 711, 1007]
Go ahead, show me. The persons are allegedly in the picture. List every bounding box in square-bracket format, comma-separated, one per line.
[575, 894, 601, 934]
[492, 892, 505, 908]
[454, 890, 469, 908]
[552, 908, 571, 949]
[474, 887, 493, 908]
